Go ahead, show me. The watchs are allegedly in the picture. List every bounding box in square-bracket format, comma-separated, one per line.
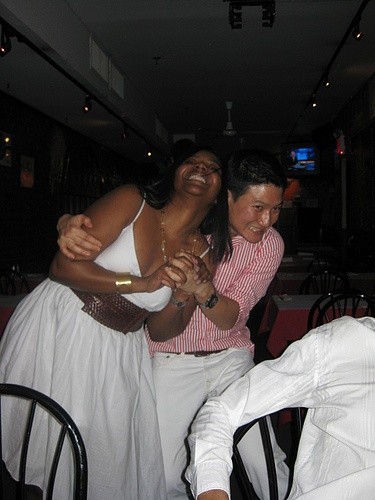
[195, 293, 218, 310]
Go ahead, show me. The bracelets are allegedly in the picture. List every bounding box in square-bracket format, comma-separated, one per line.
[115, 271, 132, 294]
[170, 295, 188, 309]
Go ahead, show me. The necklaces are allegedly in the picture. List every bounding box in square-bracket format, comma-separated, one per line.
[160, 210, 199, 271]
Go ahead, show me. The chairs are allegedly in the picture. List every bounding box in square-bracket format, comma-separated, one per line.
[0, 383, 88, 500]
[230, 407, 307, 500]
[302, 254, 375, 332]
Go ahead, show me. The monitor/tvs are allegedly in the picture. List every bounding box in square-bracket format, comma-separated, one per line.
[281, 142, 324, 178]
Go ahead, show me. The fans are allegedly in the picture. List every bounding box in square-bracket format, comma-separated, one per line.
[220, 100, 281, 137]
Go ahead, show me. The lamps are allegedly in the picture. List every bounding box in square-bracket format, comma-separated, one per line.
[311, 96, 317, 108]
[322, 75, 331, 88]
[351, 21, 363, 40]
[121, 124, 129, 139]
[0, 30, 11, 56]
[82, 95, 91, 113]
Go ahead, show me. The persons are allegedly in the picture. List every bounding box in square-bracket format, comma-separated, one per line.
[0, 141, 233, 500]
[282, 173, 301, 202]
[186, 313, 375, 500]
[57, 150, 288, 500]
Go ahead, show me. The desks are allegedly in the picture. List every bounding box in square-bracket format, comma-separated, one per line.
[265, 270, 375, 300]
[260, 293, 367, 357]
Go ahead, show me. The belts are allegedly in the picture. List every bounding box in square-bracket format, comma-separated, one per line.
[171, 348, 229, 357]
